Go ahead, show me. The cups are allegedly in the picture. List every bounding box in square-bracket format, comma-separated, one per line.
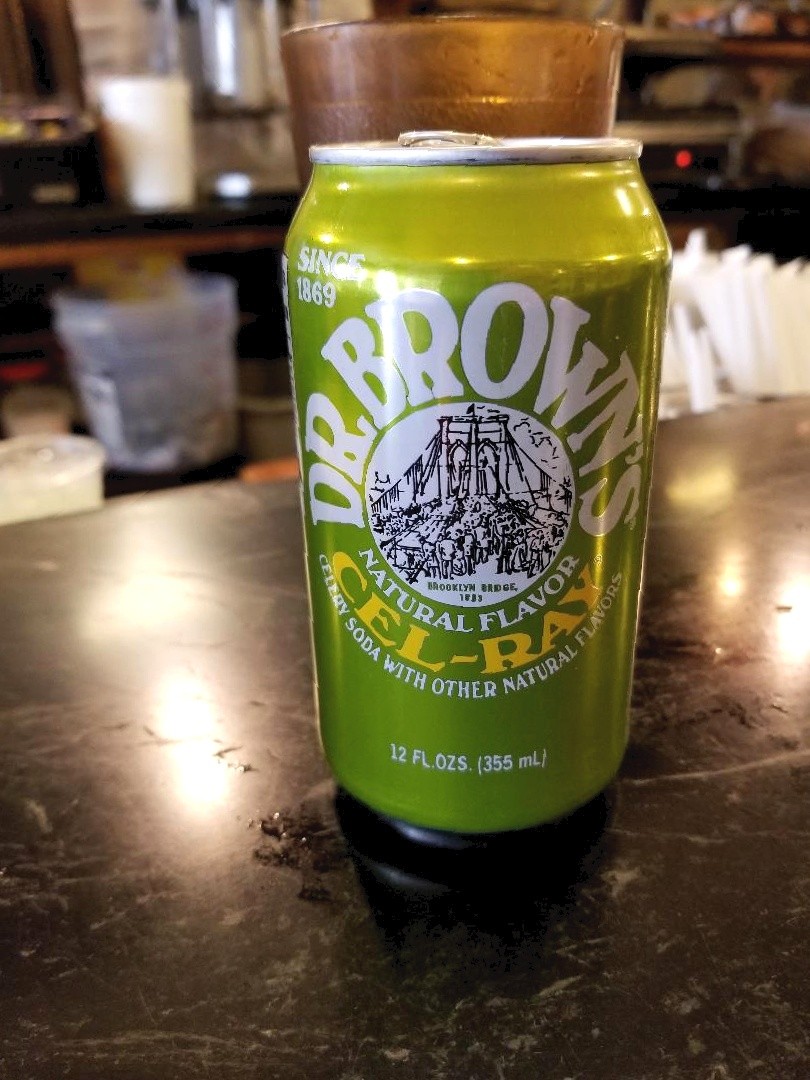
[102, 74, 196, 215]
[277, 10, 623, 206]
[1, 429, 108, 529]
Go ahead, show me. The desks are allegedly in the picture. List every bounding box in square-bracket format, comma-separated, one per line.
[0, 195, 306, 261]
[1, 394, 808, 1080]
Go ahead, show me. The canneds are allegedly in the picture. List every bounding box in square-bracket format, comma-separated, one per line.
[275, 126, 672, 836]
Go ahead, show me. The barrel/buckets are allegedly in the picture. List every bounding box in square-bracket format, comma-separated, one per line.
[56, 266, 247, 477]
